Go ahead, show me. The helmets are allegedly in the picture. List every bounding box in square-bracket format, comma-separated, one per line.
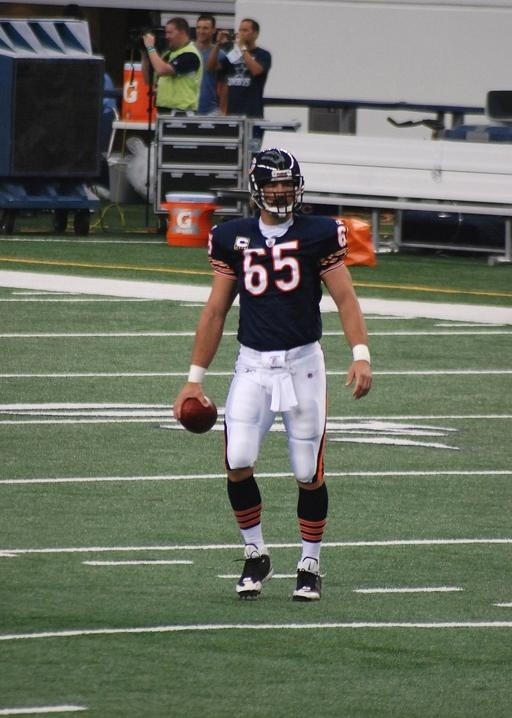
[248, 149, 307, 217]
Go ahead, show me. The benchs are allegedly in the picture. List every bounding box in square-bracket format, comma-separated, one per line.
[260, 130, 511, 265]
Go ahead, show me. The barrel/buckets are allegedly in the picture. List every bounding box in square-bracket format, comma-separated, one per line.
[122, 62, 158, 123]
[160, 192, 223, 247]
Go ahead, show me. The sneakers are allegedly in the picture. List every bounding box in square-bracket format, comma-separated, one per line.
[295, 564, 324, 600]
[237, 553, 273, 597]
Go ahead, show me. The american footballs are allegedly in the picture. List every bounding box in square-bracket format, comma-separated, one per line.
[182, 395, 217, 433]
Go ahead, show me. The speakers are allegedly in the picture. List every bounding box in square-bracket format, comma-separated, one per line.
[0, 17, 105, 207]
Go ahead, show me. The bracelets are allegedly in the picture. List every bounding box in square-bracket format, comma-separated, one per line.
[187, 363, 206, 384]
[351, 343, 371, 365]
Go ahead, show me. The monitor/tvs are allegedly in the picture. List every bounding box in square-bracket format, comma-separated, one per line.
[227, 32, 238, 40]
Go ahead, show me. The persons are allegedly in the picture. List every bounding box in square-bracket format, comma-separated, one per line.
[171, 144, 376, 604]
[136, 12, 273, 134]
